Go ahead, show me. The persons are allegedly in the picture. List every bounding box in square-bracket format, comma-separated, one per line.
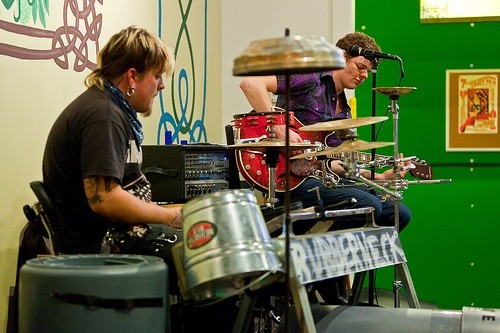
[42, 26, 184, 265]
[239, 33, 411, 306]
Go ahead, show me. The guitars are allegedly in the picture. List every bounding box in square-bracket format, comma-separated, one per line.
[235, 107, 434, 195]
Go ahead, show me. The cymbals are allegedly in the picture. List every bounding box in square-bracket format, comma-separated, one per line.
[223, 138, 321, 155]
[370, 86, 419, 98]
[298, 116, 390, 132]
[231, 111, 295, 128]
[327, 139, 396, 154]
[288, 149, 341, 160]
[232, 36, 347, 76]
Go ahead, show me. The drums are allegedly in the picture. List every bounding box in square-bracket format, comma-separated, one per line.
[180, 187, 287, 301]
[260, 200, 306, 238]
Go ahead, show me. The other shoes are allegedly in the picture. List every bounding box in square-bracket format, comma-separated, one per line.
[313, 284, 348, 305]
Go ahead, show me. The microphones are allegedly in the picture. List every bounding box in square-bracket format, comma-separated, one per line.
[349, 45, 398, 60]
